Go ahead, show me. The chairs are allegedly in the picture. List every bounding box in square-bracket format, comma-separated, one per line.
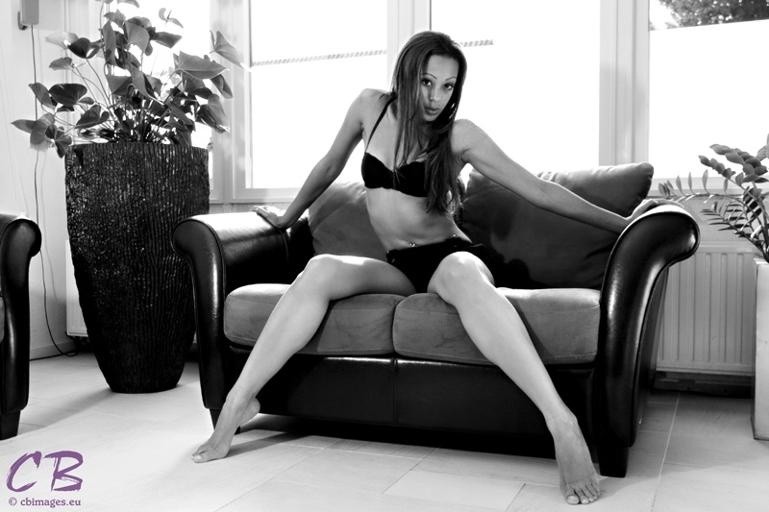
[0, 212, 43, 444]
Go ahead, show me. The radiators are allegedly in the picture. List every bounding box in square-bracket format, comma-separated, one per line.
[648, 241, 762, 381]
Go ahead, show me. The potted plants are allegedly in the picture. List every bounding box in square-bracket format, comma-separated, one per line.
[654, 132, 769, 445]
[9, 1, 248, 397]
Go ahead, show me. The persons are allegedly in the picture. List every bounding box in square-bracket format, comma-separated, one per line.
[191, 30, 685, 505]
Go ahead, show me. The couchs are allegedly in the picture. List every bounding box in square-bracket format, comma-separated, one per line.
[163, 161, 709, 479]
